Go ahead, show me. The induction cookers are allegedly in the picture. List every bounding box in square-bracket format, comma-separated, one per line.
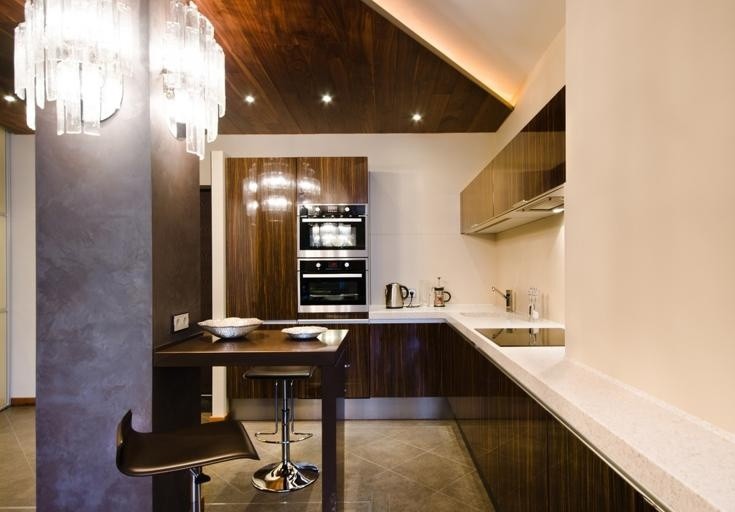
[475, 325, 565, 347]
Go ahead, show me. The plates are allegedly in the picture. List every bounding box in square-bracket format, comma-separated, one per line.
[197, 317, 264, 339]
[281, 325, 328, 341]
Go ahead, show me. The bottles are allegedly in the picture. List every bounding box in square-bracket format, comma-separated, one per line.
[434, 288, 452, 307]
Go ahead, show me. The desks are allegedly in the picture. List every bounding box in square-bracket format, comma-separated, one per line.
[152, 329, 352, 512]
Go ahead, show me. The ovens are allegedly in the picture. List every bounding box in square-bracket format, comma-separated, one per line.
[296, 203, 369, 314]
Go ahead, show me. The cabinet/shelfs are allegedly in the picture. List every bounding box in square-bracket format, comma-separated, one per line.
[367, 321, 660, 511]
[459, 87, 565, 238]
[223, 153, 366, 404]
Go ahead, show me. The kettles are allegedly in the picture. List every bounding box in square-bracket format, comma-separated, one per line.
[384, 283, 409, 309]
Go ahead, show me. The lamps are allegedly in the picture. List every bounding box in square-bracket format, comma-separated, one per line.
[12, 0, 226, 160]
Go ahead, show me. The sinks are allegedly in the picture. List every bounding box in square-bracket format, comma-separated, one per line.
[459, 312, 526, 323]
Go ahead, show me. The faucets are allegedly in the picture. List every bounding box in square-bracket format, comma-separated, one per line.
[491, 287, 514, 312]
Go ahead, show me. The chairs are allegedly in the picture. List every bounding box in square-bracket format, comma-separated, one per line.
[114, 364, 319, 512]
